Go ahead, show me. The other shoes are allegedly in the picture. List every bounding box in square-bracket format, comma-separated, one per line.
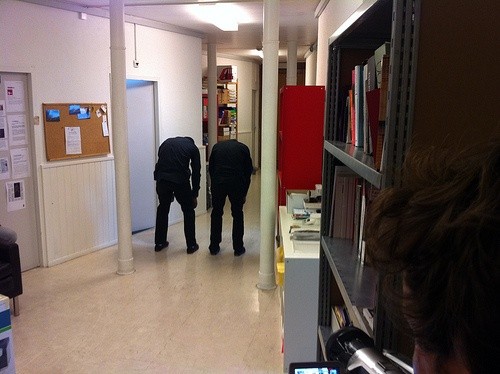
[155, 241, 169, 251]
[234, 246, 246, 256]
[187, 244, 199, 254]
[210, 246, 220, 255]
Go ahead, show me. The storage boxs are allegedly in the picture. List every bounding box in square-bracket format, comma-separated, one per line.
[286, 189, 310, 214]
[0, 293, 17, 374]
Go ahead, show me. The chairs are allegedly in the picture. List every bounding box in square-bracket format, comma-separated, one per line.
[0, 223, 23, 317]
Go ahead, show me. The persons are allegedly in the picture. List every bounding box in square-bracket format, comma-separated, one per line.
[206, 139, 254, 257]
[360, 135, 500, 374]
[151, 135, 202, 255]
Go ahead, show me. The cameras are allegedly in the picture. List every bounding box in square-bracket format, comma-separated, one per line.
[288, 327, 413, 374]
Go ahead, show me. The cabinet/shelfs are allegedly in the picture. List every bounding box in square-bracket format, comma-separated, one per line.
[278, 206, 320, 374]
[278, 85, 325, 205]
[202, 80, 238, 142]
[316, 0, 500, 374]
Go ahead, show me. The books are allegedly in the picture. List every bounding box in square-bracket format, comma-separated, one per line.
[320, 293, 374, 347]
[326, 30, 397, 271]
